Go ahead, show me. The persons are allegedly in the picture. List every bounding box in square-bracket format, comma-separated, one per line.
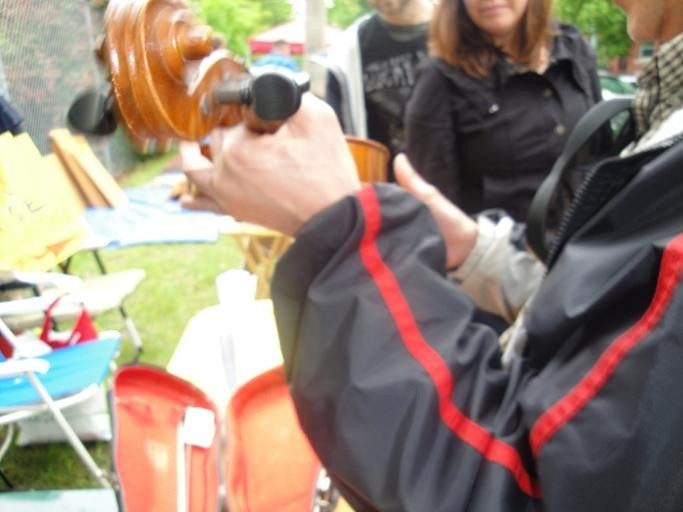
[402, 0, 618, 265]
[315, 0, 440, 183]
[177, 0, 682, 512]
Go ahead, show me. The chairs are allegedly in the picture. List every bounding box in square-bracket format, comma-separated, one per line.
[0, 296, 121, 490]
[0, 247, 147, 353]
